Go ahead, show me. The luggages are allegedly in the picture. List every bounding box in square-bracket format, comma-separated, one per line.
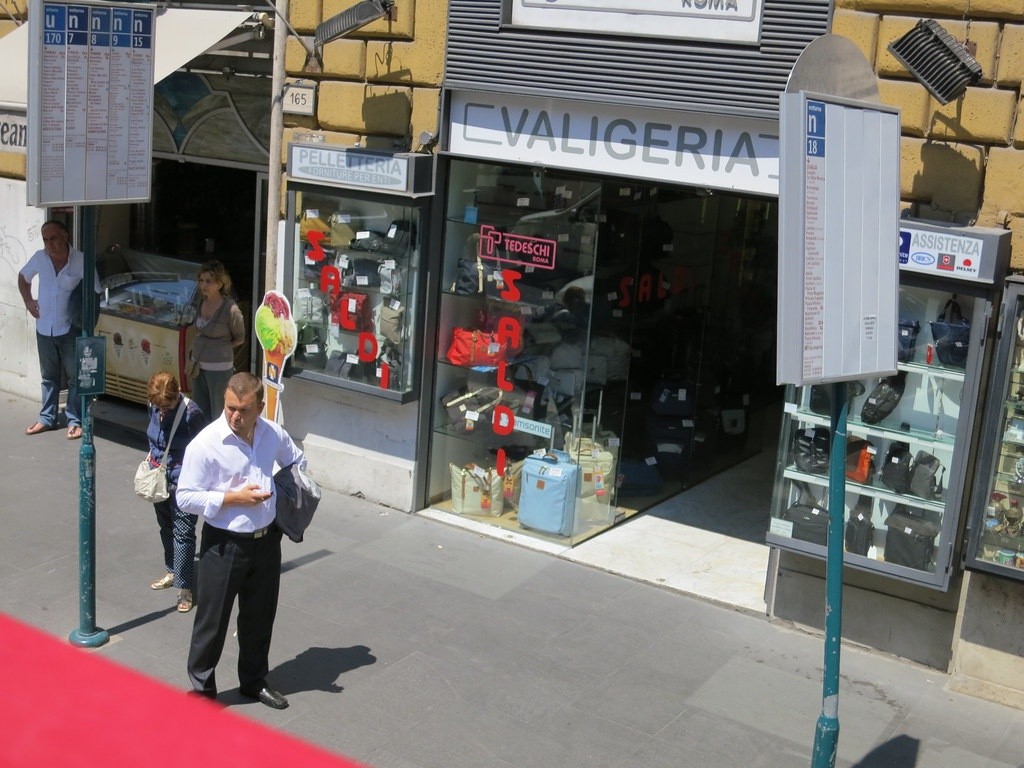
[565, 390, 617, 526]
[518, 424, 582, 537]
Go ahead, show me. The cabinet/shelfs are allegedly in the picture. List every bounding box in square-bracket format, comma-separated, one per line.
[962, 276, 1024, 583]
[431, 216, 669, 449]
[764, 214, 1012, 592]
[279, 181, 432, 404]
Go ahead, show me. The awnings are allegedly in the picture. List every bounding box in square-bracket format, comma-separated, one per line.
[0, 8, 256, 112]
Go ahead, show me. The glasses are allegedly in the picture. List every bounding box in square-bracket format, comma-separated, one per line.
[153, 398, 176, 410]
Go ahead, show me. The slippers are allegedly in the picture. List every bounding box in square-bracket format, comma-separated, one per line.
[25, 422, 53, 434]
[67, 425, 81, 440]
[151, 573, 174, 589]
[177, 591, 192, 611]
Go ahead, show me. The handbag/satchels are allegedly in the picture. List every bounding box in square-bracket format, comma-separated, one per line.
[288, 205, 414, 391]
[448, 464, 503, 516]
[450, 233, 507, 296]
[476, 310, 524, 358]
[183, 349, 200, 378]
[518, 331, 628, 399]
[466, 364, 548, 422]
[445, 327, 508, 368]
[788, 285, 971, 571]
[440, 386, 503, 433]
[134, 460, 169, 503]
[484, 442, 530, 513]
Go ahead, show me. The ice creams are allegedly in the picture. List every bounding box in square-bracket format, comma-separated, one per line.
[255, 293, 295, 420]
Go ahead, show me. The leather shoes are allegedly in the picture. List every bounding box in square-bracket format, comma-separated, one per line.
[254, 687, 288, 709]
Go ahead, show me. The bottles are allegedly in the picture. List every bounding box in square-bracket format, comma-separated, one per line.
[1007, 500, 1019, 532]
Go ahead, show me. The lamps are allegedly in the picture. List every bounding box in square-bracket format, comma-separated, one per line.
[314, 0, 397, 48]
[885, 17, 984, 105]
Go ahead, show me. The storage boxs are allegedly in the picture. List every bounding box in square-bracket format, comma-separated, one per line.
[471, 164, 583, 225]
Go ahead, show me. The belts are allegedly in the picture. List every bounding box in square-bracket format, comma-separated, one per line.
[205, 523, 273, 539]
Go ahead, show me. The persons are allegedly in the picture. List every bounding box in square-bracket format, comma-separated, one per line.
[17, 220, 103, 440]
[190, 260, 245, 423]
[175, 373, 307, 710]
[147, 369, 210, 612]
